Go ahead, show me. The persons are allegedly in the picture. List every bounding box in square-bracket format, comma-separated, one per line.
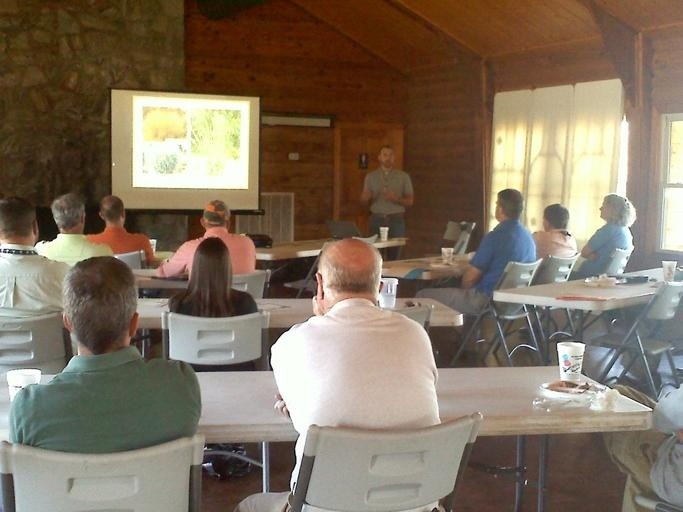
[569, 194, 637, 281]
[529, 203, 579, 281]
[360, 145, 414, 259]
[166, 237, 260, 370]
[231, 239, 450, 512]
[155, 200, 256, 276]
[603, 382, 682, 512]
[412, 189, 537, 362]
[35, 193, 116, 265]
[8, 257, 201, 457]
[0, 195, 76, 318]
[84, 195, 160, 267]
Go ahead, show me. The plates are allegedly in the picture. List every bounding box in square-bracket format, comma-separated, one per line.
[538, 383, 610, 400]
[604, 276, 649, 284]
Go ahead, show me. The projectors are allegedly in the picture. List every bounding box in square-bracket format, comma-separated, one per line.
[241, 232, 273, 248]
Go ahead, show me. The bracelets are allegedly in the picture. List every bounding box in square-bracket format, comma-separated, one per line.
[397, 194, 401, 205]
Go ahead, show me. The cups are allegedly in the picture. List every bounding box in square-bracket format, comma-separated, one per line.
[441, 248, 453, 264]
[380, 278, 398, 308]
[661, 260, 677, 281]
[380, 227, 388, 241]
[6, 368, 42, 402]
[558, 341, 584, 380]
[148, 239, 156, 254]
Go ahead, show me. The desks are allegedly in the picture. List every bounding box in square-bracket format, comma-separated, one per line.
[255, 236, 406, 274]
[1, 366, 654, 511]
